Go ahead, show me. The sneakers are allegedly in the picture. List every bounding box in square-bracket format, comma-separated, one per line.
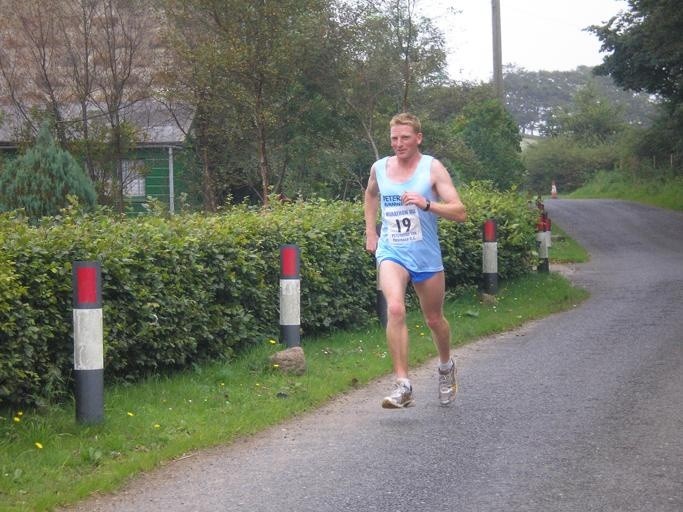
[437, 357, 458, 405]
[381, 378, 414, 408]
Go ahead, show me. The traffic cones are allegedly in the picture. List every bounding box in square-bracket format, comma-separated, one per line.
[550, 180, 558, 199]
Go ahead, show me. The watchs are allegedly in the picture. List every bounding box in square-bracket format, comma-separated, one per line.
[420, 199, 431, 212]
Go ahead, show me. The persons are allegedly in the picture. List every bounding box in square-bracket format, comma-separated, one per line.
[364, 111, 467, 409]
